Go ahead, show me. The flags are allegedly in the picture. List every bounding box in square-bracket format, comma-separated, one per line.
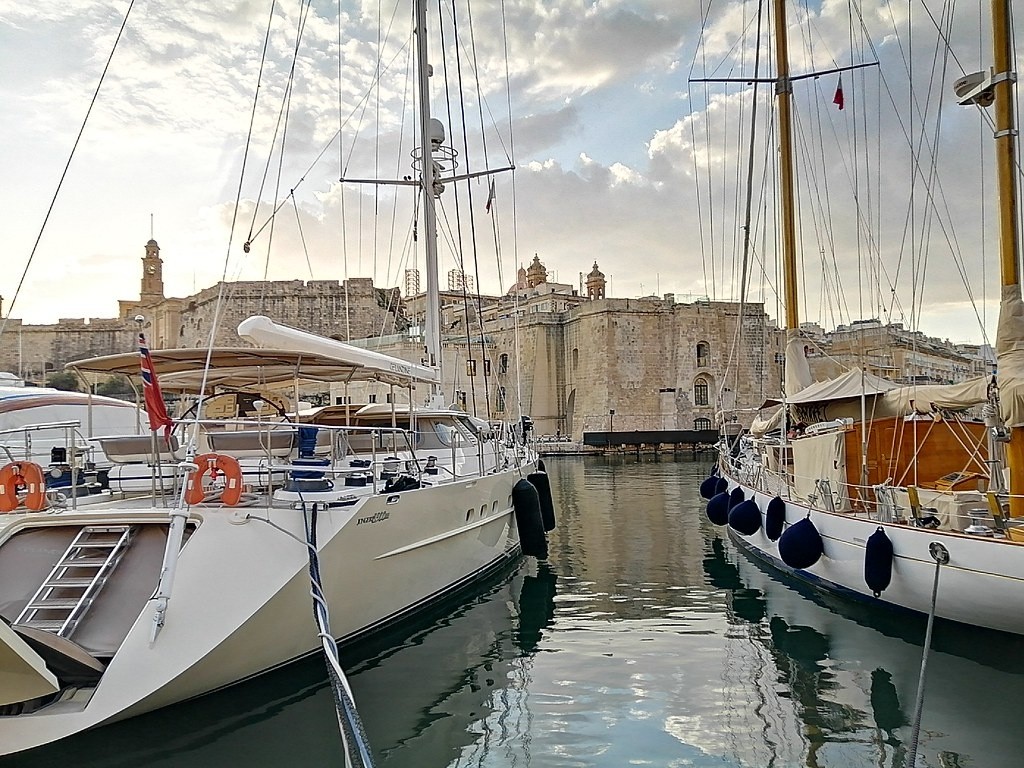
[136, 333, 171, 444]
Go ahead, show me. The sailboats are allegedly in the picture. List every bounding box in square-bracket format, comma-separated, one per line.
[688, 0, 1024, 636]
[0, 0, 556, 759]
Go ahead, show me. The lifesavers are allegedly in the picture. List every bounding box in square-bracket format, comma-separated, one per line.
[184, 452, 243, 505]
[0, 461, 45, 513]
